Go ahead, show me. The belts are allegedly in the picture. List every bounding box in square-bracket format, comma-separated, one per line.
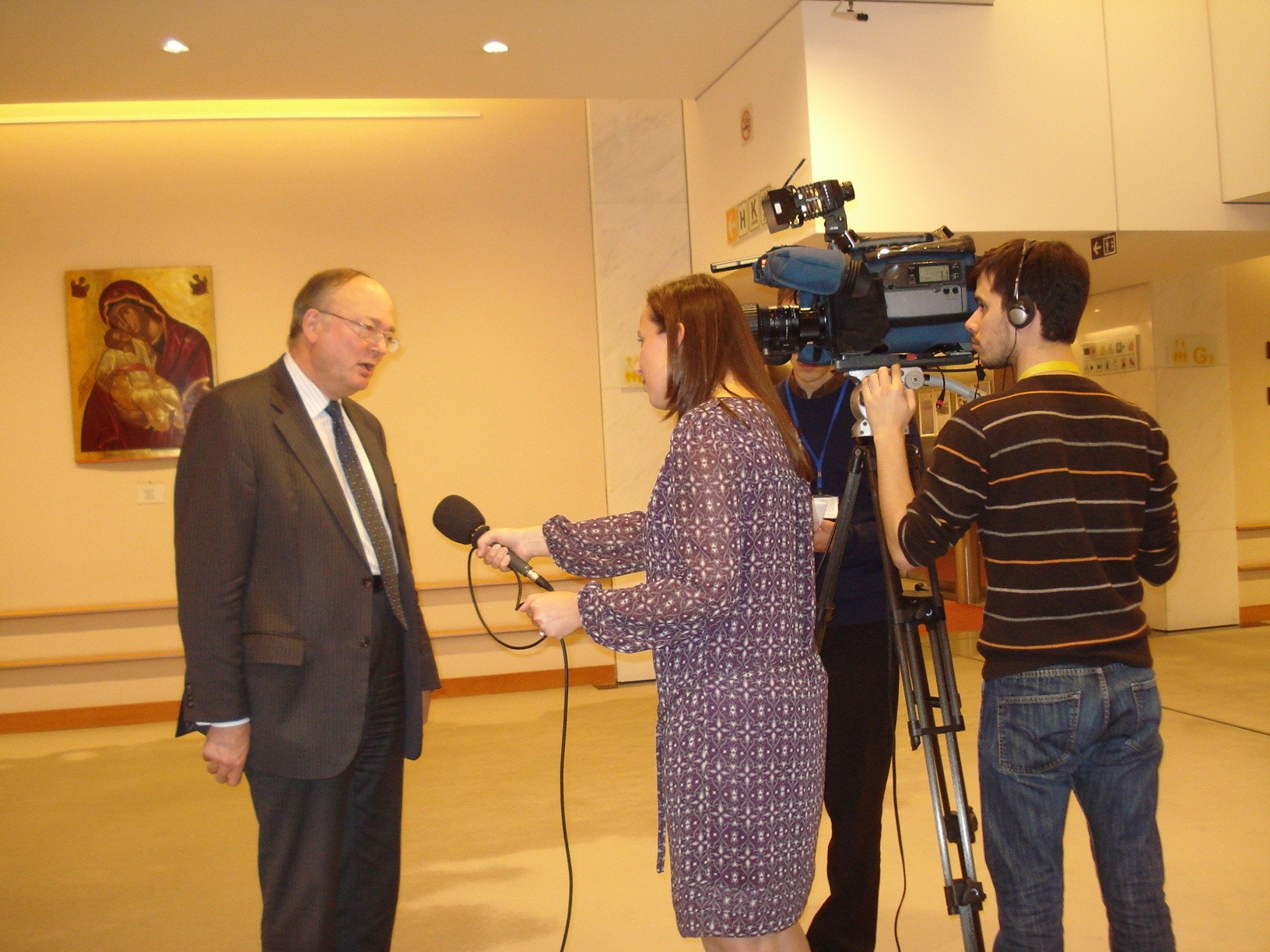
[365, 569, 394, 599]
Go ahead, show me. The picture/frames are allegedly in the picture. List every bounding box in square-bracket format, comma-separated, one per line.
[917, 381, 992, 439]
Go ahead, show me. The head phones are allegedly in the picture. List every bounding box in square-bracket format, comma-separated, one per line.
[1005, 239, 1036, 329]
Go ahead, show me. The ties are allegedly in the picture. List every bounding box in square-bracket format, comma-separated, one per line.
[323, 401, 412, 633]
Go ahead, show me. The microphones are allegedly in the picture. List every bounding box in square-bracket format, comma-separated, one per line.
[433, 493, 556, 592]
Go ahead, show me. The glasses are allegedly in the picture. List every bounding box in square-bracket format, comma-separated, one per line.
[313, 306, 399, 351]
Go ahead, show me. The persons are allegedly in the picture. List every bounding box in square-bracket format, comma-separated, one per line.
[176, 270, 444, 952]
[476, 274, 830, 952]
[862, 239, 1182, 952]
[773, 354, 925, 952]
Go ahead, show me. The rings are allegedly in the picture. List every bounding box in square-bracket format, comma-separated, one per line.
[538, 629, 545, 636]
